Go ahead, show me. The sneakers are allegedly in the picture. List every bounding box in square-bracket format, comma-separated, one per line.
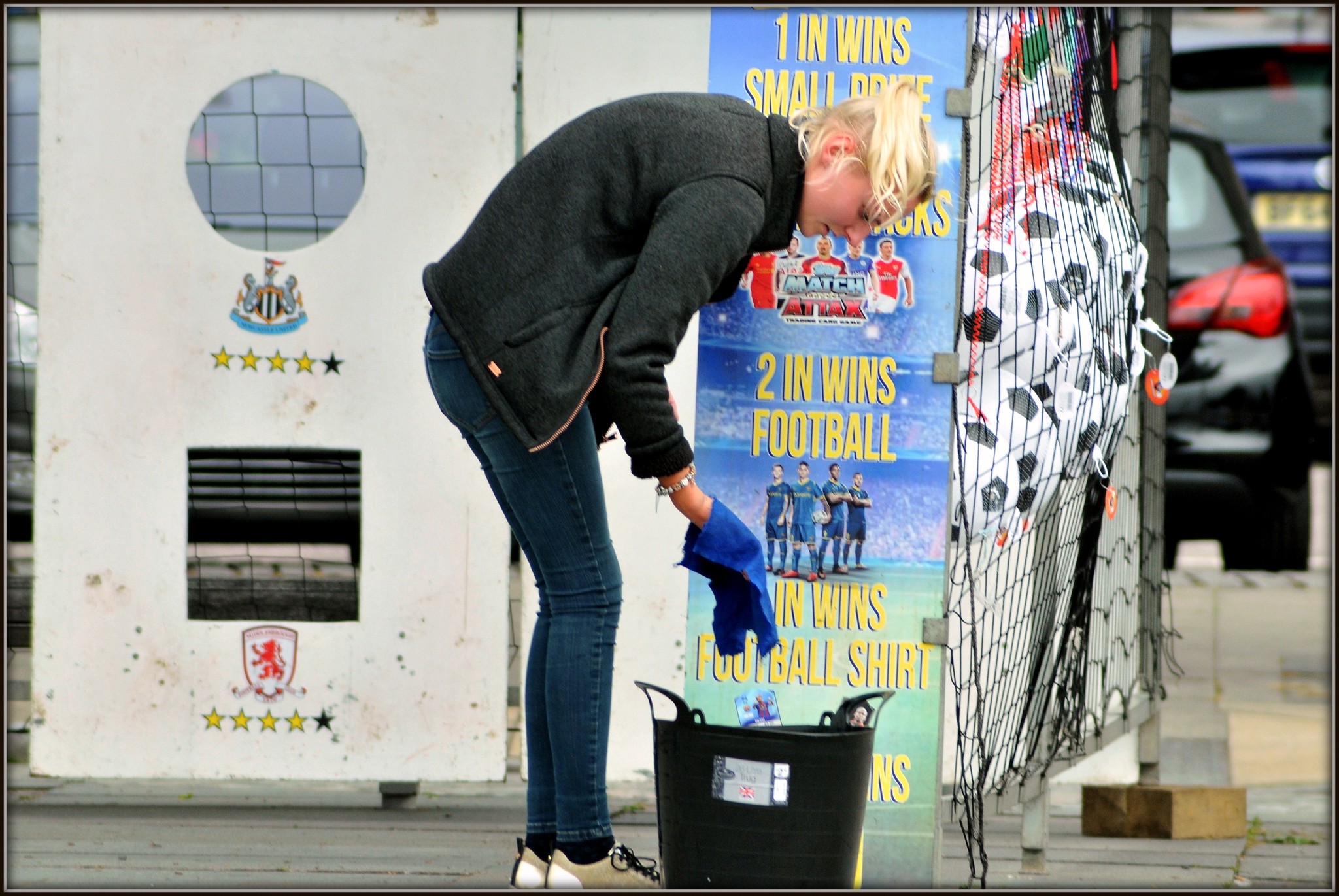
[774, 568, 784, 576]
[833, 565, 848, 575]
[856, 563, 866, 569]
[765, 565, 772, 571]
[807, 571, 817, 581]
[546, 838, 662, 889]
[817, 568, 826, 578]
[781, 570, 799, 578]
[842, 563, 848, 572]
[510, 837, 551, 889]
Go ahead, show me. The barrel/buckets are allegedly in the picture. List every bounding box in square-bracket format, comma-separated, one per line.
[634, 680, 896, 889]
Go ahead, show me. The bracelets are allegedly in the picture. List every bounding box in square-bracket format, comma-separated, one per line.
[655, 461, 695, 496]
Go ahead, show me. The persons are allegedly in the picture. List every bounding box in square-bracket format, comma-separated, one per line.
[839, 240, 880, 313]
[421, 82, 937, 888]
[774, 236, 807, 308]
[842, 471, 872, 572]
[741, 252, 778, 309]
[760, 464, 791, 575]
[782, 461, 830, 582]
[868, 239, 913, 313]
[801, 237, 848, 275]
[817, 463, 853, 579]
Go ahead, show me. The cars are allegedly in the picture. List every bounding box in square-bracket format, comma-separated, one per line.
[1169, 10, 1337, 462]
[1163, 124, 1318, 570]
